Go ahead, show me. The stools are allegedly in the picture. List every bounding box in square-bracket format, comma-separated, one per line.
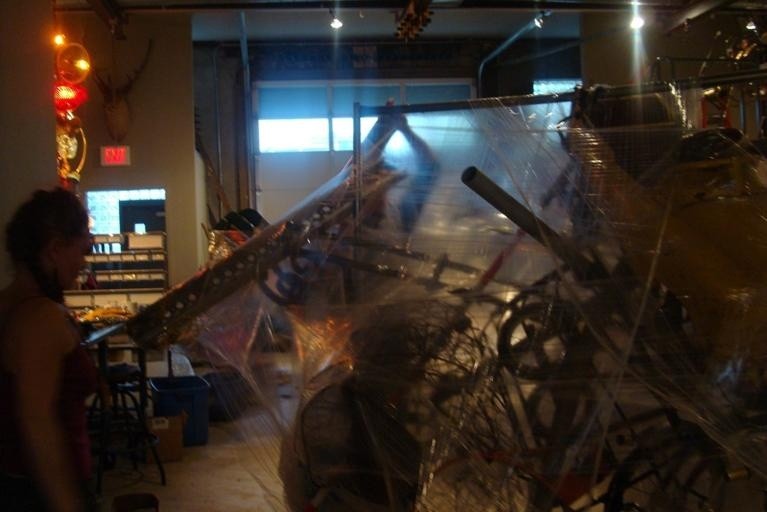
[86, 361, 172, 498]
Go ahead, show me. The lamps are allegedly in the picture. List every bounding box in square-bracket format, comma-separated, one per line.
[535, 12, 549, 32]
[330, 8, 344, 31]
[394, 0, 436, 46]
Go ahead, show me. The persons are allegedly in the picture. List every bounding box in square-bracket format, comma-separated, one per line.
[0, 189, 109, 511]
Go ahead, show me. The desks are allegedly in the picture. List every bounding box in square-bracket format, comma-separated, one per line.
[86, 332, 154, 473]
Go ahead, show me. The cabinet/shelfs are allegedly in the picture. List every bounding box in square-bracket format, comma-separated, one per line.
[61, 229, 172, 318]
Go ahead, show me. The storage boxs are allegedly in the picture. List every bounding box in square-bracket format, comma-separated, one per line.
[202, 370, 249, 421]
[149, 372, 212, 452]
[144, 412, 187, 462]
